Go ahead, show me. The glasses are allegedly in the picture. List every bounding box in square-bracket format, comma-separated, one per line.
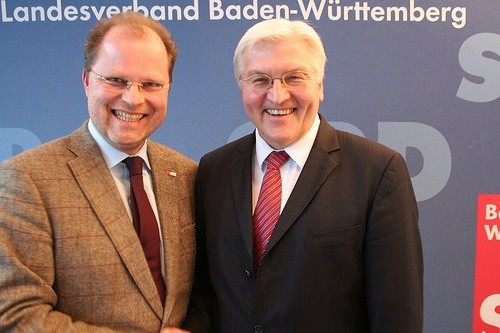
[90, 69, 169, 97]
[243, 71, 316, 91]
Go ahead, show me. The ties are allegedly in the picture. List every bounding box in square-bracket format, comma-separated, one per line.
[252, 151, 290, 278]
[121, 156, 166, 311]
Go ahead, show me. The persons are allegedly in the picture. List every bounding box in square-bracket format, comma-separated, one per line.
[0, 12, 200, 333]
[183, 18, 426, 333]
